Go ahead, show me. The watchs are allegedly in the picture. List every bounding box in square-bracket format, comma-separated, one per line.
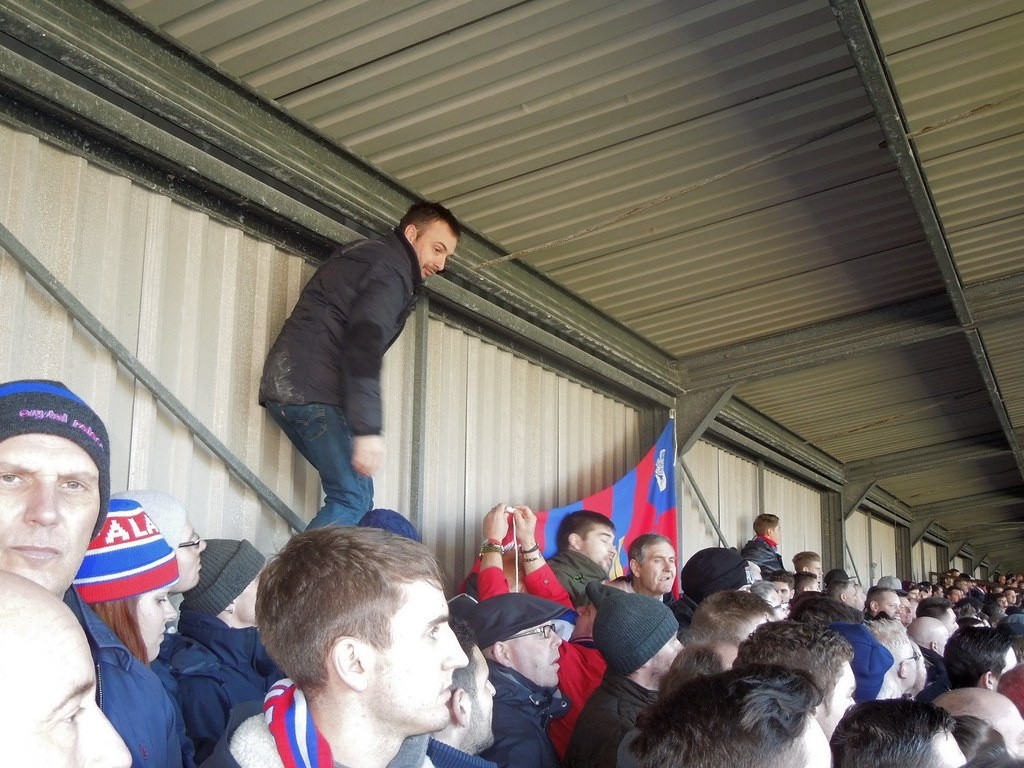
[478, 544, 504, 561]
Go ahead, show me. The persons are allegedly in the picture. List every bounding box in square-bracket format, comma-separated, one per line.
[259, 203, 458, 532]
[0, 379, 1024, 767]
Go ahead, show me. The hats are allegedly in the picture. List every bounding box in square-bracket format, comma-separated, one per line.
[72, 499, 180, 603]
[586, 580, 627, 611]
[828, 623, 894, 703]
[919, 585, 928, 593]
[467, 593, 570, 651]
[998, 613, 1024, 636]
[593, 593, 679, 675]
[181, 539, 265, 616]
[0, 379, 110, 541]
[682, 548, 748, 605]
[357, 509, 419, 544]
[111, 490, 186, 549]
[877, 575, 908, 595]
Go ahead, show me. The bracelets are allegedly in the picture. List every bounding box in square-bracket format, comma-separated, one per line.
[520, 543, 539, 555]
[523, 553, 543, 563]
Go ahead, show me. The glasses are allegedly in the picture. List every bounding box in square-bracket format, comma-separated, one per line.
[908, 652, 920, 660]
[492, 623, 555, 653]
[179, 534, 200, 549]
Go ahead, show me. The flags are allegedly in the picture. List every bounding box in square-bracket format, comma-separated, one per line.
[460, 414, 679, 602]
